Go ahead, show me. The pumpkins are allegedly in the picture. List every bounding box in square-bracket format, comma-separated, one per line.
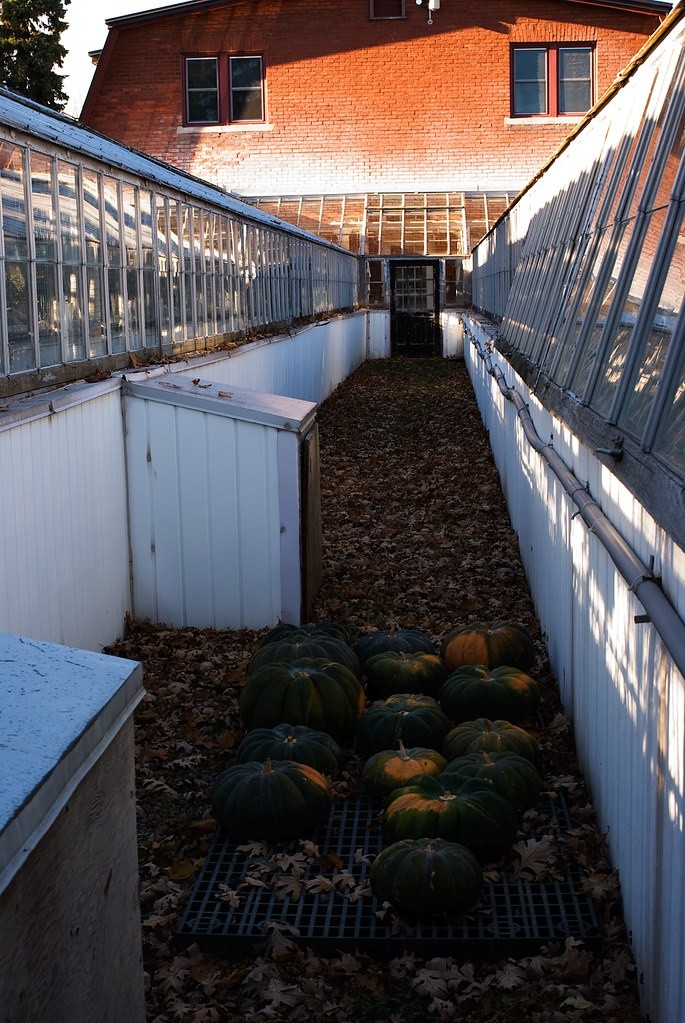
[207, 620, 544, 926]
